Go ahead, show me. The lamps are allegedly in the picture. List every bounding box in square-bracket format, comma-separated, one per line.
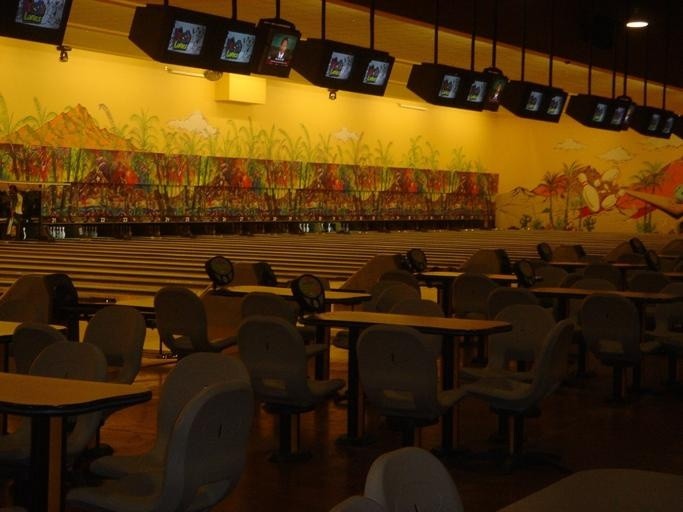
[620, 0, 657, 33]
